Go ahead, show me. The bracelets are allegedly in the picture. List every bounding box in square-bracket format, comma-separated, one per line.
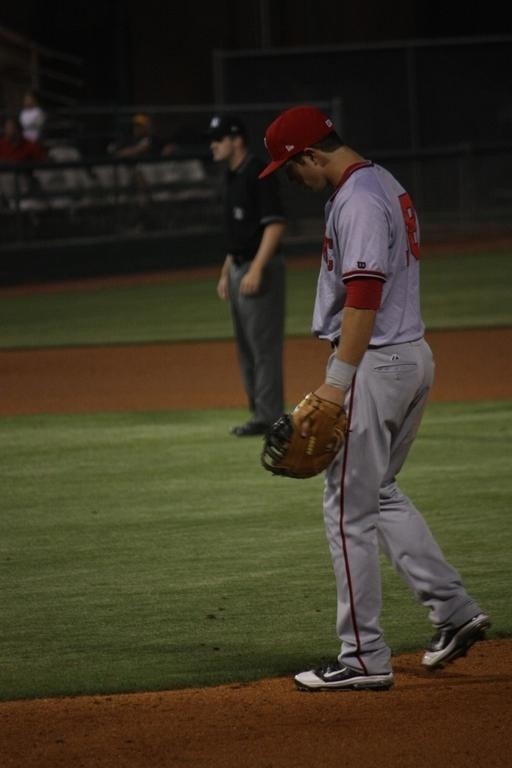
[324, 357, 359, 392]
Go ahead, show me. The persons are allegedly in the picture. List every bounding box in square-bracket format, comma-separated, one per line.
[257, 106, 493, 694]
[18, 88, 49, 145]
[199, 111, 291, 436]
[1, 116, 55, 210]
[110, 111, 184, 234]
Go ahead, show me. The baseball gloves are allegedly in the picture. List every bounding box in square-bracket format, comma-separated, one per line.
[261, 392, 348, 478]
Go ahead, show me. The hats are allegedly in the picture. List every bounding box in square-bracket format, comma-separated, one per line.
[203, 113, 241, 140]
[258, 104, 335, 179]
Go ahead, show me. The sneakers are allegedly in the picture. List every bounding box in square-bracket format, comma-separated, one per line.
[292, 659, 394, 693]
[230, 421, 262, 437]
[421, 611, 490, 672]
